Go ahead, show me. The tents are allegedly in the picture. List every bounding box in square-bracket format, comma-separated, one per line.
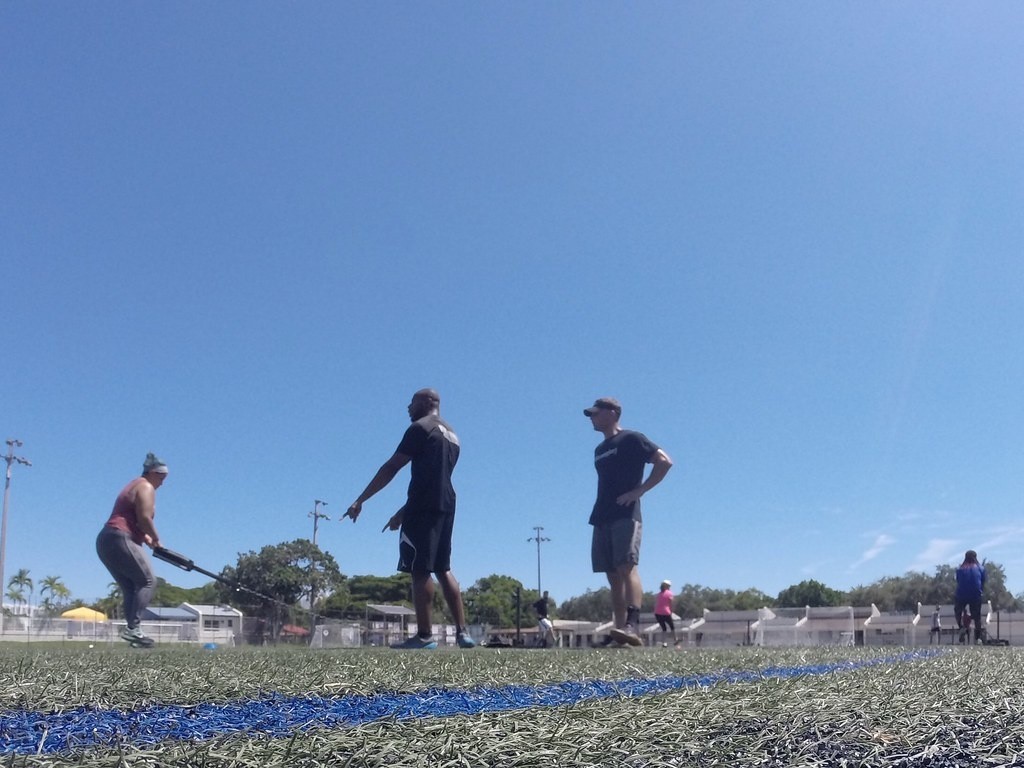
[139, 606, 197, 623]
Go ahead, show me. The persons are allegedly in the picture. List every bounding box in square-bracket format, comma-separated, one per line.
[929, 604, 941, 645]
[962, 609, 973, 645]
[582, 397, 673, 647]
[95, 453, 172, 647]
[529, 591, 560, 648]
[954, 549, 988, 645]
[654, 579, 682, 648]
[338, 386, 477, 650]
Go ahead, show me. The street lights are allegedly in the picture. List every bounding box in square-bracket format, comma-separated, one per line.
[527, 527, 550, 600]
[308, 499, 332, 612]
[0, 438, 32, 633]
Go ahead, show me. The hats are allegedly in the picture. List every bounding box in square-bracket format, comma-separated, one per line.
[584, 398, 621, 416]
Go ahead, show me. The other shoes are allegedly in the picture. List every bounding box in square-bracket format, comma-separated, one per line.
[592, 637, 627, 648]
[977, 639, 982, 645]
[611, 626, 641, 646]
[959, 628, 966, 642]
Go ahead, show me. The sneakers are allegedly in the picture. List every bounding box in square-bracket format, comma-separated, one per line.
[122, 626, 152, 645]
[458, 633, 475, 648]
[131, 638, 154, 648]
[391, 637, 436, 649]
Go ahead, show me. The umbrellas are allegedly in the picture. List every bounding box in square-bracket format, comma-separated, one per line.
[61, 606, 106, 636]
